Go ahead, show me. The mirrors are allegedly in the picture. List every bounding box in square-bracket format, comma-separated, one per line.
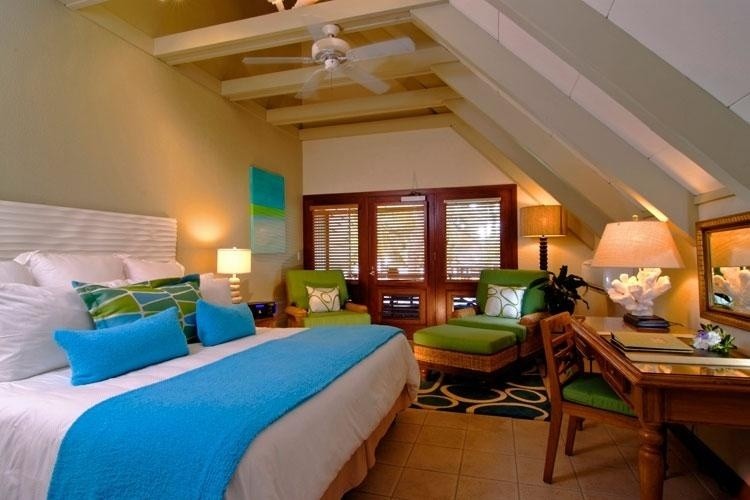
[695, 210, 750, 331]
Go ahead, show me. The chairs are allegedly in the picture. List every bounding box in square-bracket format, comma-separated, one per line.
[536, 312, 642, 484]
[448, 270, 551, 358]
[283, 267, 371, 328]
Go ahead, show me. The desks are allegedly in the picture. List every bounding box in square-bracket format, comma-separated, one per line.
[571, 314, 750, 500]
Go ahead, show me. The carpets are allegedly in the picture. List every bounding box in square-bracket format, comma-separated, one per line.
[409, 358, 551, 422]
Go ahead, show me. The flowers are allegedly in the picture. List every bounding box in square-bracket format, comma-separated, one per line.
[691, 321, 738, 354]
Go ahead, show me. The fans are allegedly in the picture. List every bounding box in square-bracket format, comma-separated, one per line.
[241, 23, 418, 101]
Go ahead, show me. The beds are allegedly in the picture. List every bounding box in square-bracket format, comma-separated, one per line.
[0, 200, 419, 500]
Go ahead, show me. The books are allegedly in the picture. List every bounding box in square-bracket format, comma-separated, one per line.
[610, 332, 695, 354]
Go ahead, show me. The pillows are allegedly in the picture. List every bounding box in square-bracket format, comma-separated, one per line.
[484, 284, 527, 318]
[306, 283, 340, 313]
[0, 249, 256, 386]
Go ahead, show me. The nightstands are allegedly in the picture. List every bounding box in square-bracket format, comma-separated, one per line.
[255, 316, 278, 328]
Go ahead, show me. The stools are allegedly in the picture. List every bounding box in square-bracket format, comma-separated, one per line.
[413, 324, 518, 393]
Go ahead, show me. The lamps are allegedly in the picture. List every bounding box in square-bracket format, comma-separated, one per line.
[590, 219, 686, 328]
[520, 204, 570, 270]
[709, 228, 750, 320]
